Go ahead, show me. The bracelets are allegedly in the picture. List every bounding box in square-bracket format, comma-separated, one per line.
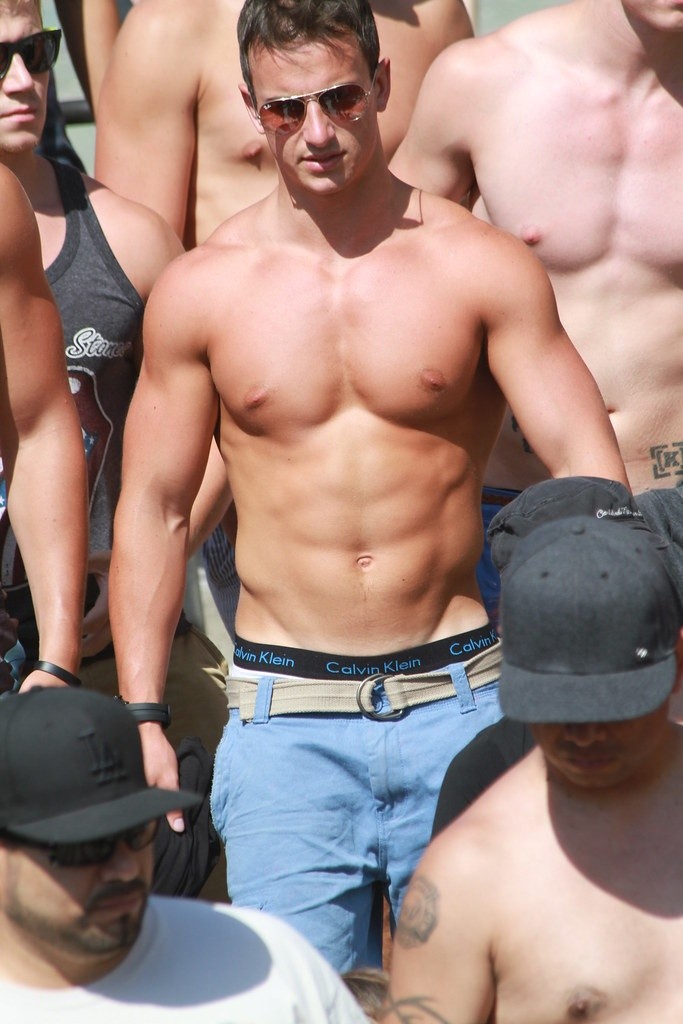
[128, 702, 172, 727]
[33, 660, 82, 688]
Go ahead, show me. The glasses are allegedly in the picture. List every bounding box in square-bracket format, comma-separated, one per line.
[0, 28, 62, 79]
[245, 62, 380, 135]
[0, 816, 162, 866]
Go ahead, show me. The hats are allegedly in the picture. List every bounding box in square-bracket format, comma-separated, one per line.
[486, 475, 671, 575]
[0, 687, 204, 844]
[497, 519, 683, 724]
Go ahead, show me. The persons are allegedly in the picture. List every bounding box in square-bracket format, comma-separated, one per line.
[0, 689, 369, 1024]
[93, 0, 633, 972]
[431, 476, 642, 836]
[32, 0, 133, 170]
[384, 517, 682, 1024]
[0, 0, 231, 680]
[0, 163, 88, 691]
[386, 0, 683, 628]
